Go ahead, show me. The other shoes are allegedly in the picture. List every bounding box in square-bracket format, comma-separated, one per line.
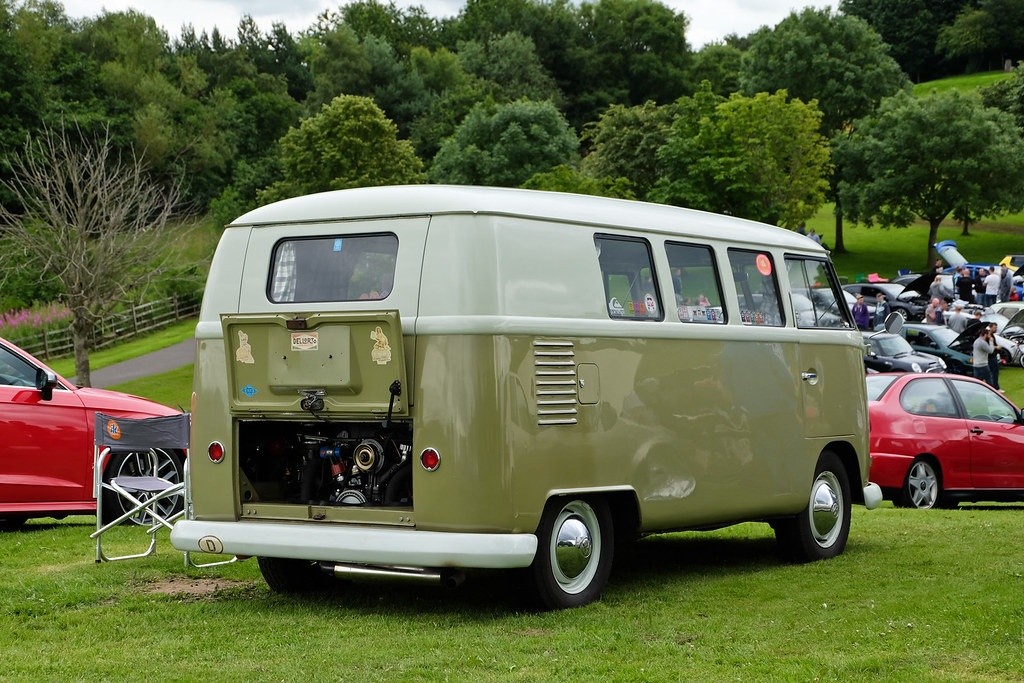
[997, 389, 1005, 393]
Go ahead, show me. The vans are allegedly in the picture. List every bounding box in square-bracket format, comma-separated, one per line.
[168, 184, 883, 604]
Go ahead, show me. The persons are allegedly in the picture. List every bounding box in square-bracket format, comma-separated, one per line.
[925, 299, 947, 326]
[983, 267, 1000, 306]
[948, 305, 967, 333]
[874, 293, 890, 330]
[986, 323, 1005, 393]
[933, 259, 944, 273]
[807, 228, 820, 243]
[954, 267, 977, 304]
[967, 312, 982, 327]
[975, 269, 987, 305]
[928, 275, 951, 298]
[973, 329, 994, 386]
[696, 295, 711, 306]
[851, 294, 869, 329]
[798, 221, 806, 235]
[673, 268, 683, 305]
[1000, 263, 1011, 302]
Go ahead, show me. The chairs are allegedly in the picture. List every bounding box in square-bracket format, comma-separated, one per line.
[347, 250, 396, 301]
[595, 265, 729, 319]
[90, 410, 238, 568]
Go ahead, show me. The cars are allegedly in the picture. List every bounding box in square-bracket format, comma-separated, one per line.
[861, 331, 950, 374]
[734, 292, 845, 327]
[902, 321, 1004, 377]
[790, 287, 877, 325]
[839, 241, 1024, 321]
[0, 338, 190, 531]
[865, 373, 1024, 508]
[922, 301, 1024, 368]
